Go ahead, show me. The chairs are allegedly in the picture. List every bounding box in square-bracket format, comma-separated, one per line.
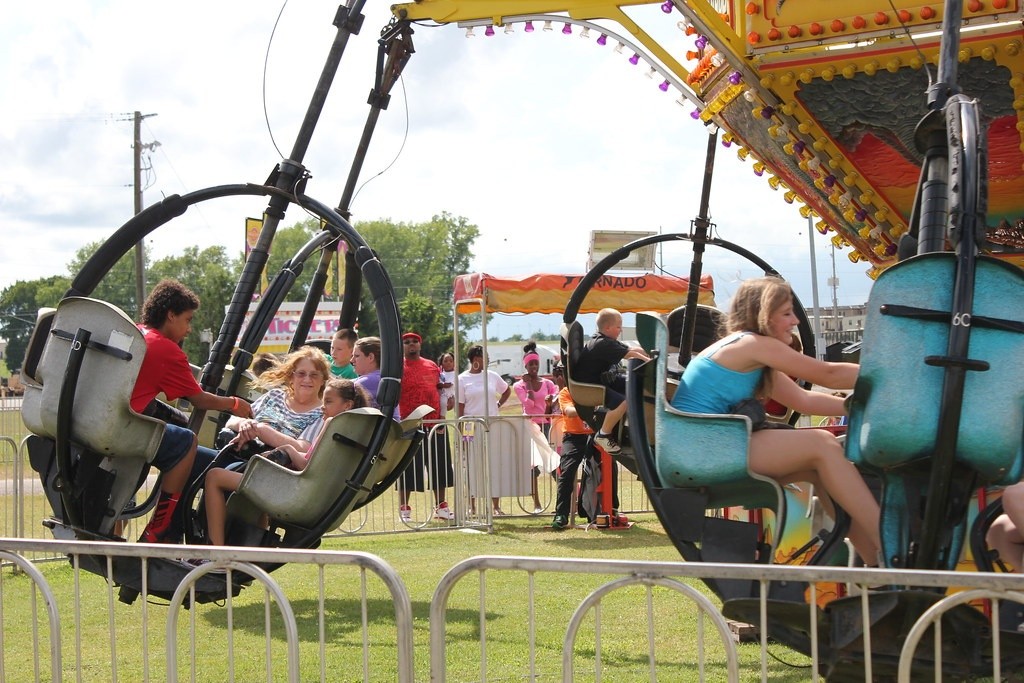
[564, 322, 625, 445]
[629, 251, 1024, 611]
[153, 355, 437, 560]
[31, 295, 169, 533]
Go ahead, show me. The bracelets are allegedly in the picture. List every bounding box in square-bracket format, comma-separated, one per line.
[497, 400, 501, 406]
[229, 395, 239, 413]
[527, 389, 534, 393]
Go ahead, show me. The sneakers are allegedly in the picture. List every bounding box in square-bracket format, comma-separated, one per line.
[433, 501, 455, 520]
[493, 508, 504, 515]
[134, 526, 183, 563]
[551, 514, 569, 528]
[398, 505, 412, 524]
[593, 431, 622, 455]
[182, 552, 229, 575]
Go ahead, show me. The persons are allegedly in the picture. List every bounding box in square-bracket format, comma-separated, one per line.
[576, 308, 651, 452]
[434, 351, 455, 434]
[551, 362, 620, 531]
[827, 390, 848, 426]
[115, 276, 255, 543]
[671, 258, 882, 589]
[350, 336, 401, 423]
[763, 333, 802, 416]
[458, 345, 512, 515]
[395, 331, 454, 522]
[986, 481, 1024, 631]
[324, 329, 358, 378]
[514, 339, 558, 513]
[183, 345, 373, 574]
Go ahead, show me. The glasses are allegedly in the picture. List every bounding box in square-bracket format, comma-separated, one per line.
[291, 368, 322, 380]
[402, 339, 421, 344]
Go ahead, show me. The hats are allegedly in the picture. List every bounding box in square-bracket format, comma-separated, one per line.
[402, 332, 423, 340]
